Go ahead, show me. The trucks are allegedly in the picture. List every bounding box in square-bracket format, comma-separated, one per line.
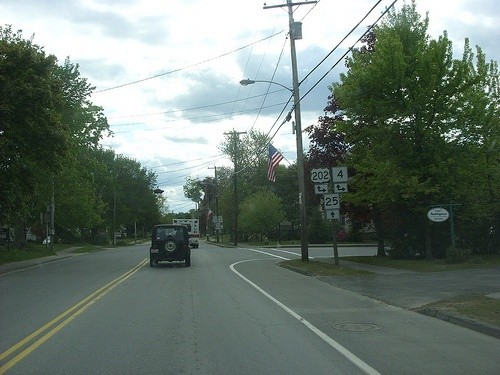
[173, 218, 200, 249]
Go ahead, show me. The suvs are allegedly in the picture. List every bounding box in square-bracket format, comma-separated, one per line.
[149, 223, 193, 268]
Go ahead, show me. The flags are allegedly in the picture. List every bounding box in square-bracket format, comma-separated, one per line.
[268, 145, 283, 182]
[208, 208, 213, 219]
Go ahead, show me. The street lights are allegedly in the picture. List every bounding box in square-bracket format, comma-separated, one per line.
[239, 78, 308, 261]
